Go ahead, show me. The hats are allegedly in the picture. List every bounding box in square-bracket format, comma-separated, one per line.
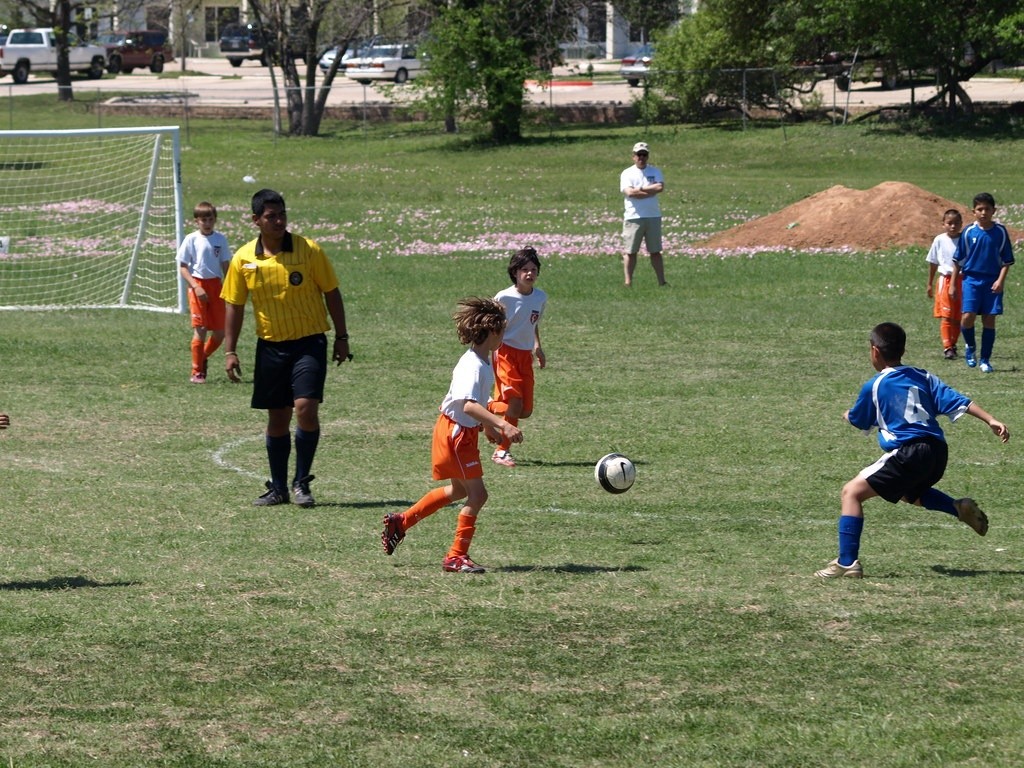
[633, 142, 649, 153]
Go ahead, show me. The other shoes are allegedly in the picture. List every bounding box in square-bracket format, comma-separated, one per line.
[944, 346, 958, 359]
[979, 358, 993, 373]
[965, 346, 977, 367]
[191, 358, 209, 383]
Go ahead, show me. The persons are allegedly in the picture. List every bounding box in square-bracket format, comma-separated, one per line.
[175, 202, 232, 385]
[219, 188, 349, 507]
[0, 414, 10, 429]
[478, 245, 548, 467]
[812, 322, 1010, 580]
[620, 142, 671, 288]
[379, 296, 524, 574]
[925, 192, 1014, 372]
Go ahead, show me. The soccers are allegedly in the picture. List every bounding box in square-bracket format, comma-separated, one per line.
[594, 453, 636, 494]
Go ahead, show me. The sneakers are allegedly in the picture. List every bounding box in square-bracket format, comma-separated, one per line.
[383, 512, 406, 556]
[291, 475, 317, 506]
[254, 480, 291, 505]
[443, 555, 486, 574]
[491, 446, 516, 466]
[812, 558, 864, 578]
[954, 498, 989, 537]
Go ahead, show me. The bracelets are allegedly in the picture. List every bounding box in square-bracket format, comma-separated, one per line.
[224, 351, 238, 357]
[335, 333, 349, 341]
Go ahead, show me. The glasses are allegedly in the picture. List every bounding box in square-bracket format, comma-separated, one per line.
[636, 152, 648, 157]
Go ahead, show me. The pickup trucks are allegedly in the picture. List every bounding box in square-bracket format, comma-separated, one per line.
[0, 28, 109, 85]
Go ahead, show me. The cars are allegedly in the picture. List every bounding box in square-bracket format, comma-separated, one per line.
[319, 42, 370, 75]
[344, 43, 432, 85]
[94, 29, 174, 74]
[620, 45, 657, 87]
[219, 22, 307, 68]
[827, 42, 975, 92]
